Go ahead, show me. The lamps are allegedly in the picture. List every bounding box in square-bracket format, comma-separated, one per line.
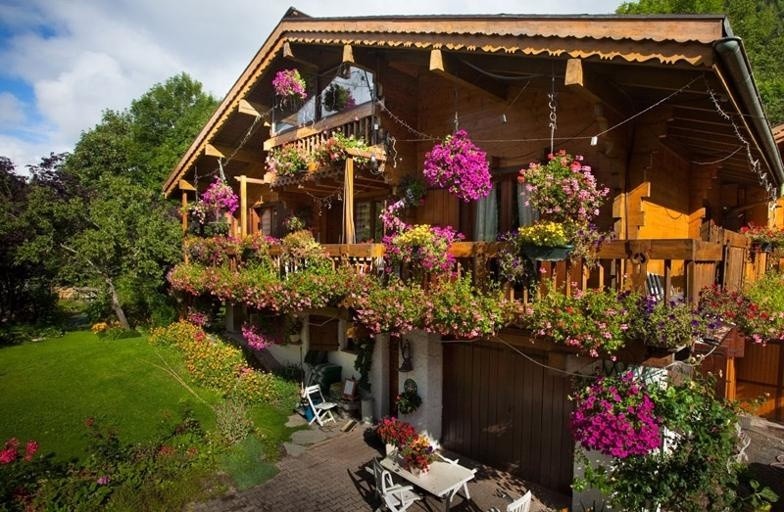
[399, 339, 413, 373]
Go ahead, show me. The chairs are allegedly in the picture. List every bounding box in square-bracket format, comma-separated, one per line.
[305, 384, 338, 427]
[485, 489, 531, 512]
[373, 456, 422, 511]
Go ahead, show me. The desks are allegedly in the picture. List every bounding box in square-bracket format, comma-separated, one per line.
[379, 446, 475, 502]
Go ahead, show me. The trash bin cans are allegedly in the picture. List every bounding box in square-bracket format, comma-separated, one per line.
[362, 397, 373, 423]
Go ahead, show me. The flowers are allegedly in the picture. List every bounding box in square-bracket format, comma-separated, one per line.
[517, 149, 610, 220]
[264, 139, 308, 178]
[273, 69, 307, 99]
[517, 221, 571, 247]
[322, 83, 357, 113]
[313, 127, 367, 164]
[422, 129, 493, 204]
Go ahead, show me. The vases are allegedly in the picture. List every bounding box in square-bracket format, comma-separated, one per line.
[522, 243, 574, 262]
[386, 443, 398, 456]
[408, 464, 430, 479]
